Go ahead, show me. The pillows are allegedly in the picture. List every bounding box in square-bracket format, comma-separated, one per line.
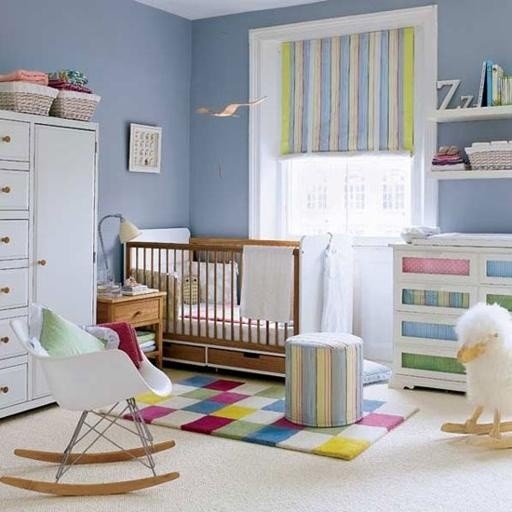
[41, 307, 105, 359]
[187, 261, 238, 306]
[131, 268, 180, 319]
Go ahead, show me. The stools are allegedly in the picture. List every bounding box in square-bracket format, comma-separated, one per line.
[284, 332, 364, 428]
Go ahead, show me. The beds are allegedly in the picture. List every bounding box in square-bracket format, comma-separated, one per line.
[120, 228, 354, 378]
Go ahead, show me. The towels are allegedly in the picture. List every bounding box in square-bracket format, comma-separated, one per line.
[240, 245, 292, 324]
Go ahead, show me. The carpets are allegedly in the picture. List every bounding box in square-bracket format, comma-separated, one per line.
[93, 374, 420, 462]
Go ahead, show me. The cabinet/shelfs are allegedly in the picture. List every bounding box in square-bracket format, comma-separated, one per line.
[388, 242, 512, 394]
[0, 109, 99, 419]
[428, 105, 512, 181]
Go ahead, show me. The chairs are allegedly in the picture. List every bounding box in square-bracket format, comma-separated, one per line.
[0, 316, 179, 497]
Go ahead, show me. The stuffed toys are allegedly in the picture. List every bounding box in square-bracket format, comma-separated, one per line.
[181, 275, 198, 305]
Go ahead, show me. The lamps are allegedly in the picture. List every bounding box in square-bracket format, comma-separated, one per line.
[98, 214, 141, 293]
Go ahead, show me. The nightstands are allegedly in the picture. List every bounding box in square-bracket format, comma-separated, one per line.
[97, 290, 167, 369]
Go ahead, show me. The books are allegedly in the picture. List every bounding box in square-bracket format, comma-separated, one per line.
[475, 59, 512, 107]
[121, 284, 159, 296]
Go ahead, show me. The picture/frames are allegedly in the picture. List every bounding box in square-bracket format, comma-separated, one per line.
[128, 123, 162, 174]
